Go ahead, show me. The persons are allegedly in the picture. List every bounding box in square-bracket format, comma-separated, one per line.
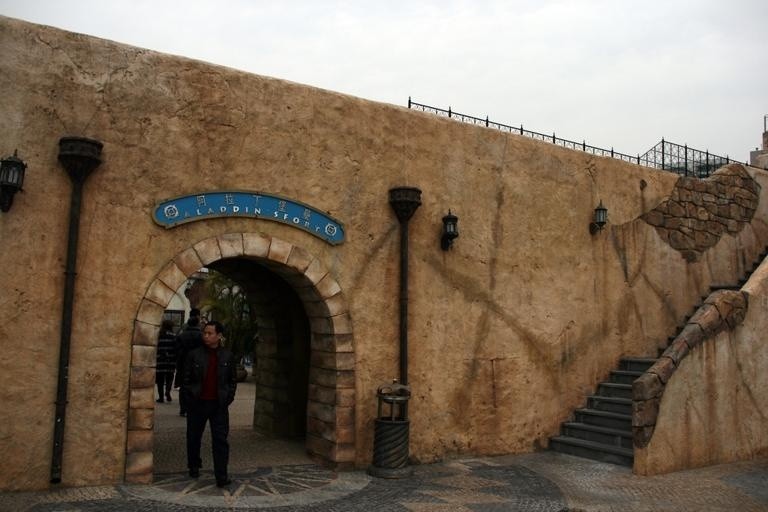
[156, 309, 237, 490]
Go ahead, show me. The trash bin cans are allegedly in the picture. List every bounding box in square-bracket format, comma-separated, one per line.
[366, 378, 413, 479]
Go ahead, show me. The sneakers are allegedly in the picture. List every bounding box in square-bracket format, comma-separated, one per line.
[165, 392, 172, 402]
[156, 398, 164, 402]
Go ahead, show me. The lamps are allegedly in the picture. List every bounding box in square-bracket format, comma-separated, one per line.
[441, 208, 458, 251]
[590, 200, 609, 235]
[1, 147, 26, 212]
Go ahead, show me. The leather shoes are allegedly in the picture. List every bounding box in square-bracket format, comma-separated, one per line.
[189, 467, 200, 478]
[217, 478, 232, 489]
